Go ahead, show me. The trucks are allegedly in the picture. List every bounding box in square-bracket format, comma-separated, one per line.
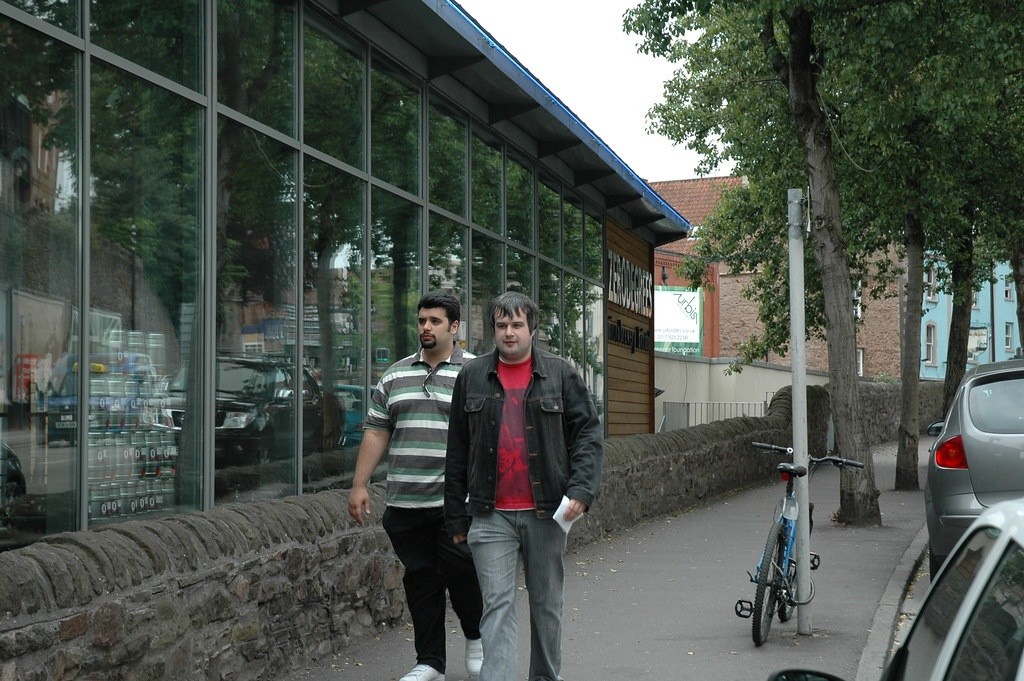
[28, 350, 158, 444]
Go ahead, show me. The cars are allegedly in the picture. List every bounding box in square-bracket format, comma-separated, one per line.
[767, 496, 1024, 680]
[0, 439, 26, 513]
[325, 383, 375, 449]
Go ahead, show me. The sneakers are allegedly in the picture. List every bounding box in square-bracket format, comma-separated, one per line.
[465, 638, 485, 676]
[400, 664, 445, 681]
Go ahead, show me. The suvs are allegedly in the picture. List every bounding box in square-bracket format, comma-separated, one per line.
[131, 360, 324, 473]
[922, 356, 1023, 582]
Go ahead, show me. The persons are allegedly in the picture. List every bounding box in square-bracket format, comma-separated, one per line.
[348, 290, 483, 681]
[441, 291, 604, 681]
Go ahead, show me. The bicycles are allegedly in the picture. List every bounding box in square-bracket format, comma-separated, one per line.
[735, 436, 865, 651]
